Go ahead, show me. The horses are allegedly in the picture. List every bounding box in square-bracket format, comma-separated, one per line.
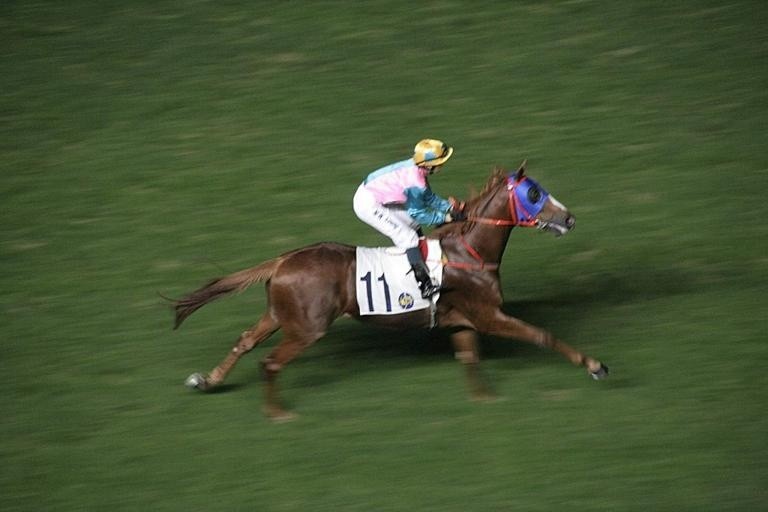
[157, 157, 609, 422]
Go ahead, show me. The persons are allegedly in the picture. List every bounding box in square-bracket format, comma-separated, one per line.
[353, 139, 456, 298]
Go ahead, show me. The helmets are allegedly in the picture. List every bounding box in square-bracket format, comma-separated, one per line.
[413, 139, 453, 166]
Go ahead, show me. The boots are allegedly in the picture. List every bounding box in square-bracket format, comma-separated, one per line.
[406, 248, 443, 298]
